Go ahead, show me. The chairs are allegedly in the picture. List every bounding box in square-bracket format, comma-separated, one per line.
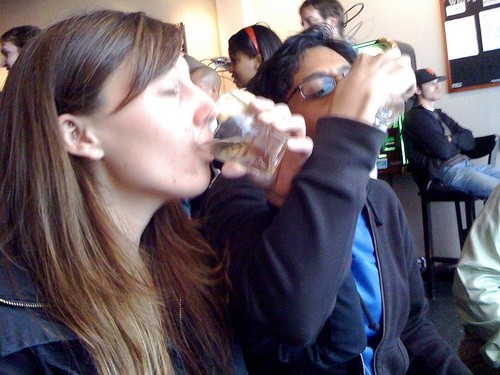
[407, 134, 496, 301]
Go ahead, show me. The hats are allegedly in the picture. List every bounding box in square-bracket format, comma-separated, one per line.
[414, 68, 447, 85]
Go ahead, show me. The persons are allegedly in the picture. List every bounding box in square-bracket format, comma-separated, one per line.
[0, 25, 44, 72]
[190, 65, 221, 104]
[227, 21, 283, 89]
[0, 8, 315, 375]
[200, 21, 474, 375]
[299, 0, 344, 41]
[402, 68, 500, 204]
[451, 182, 500, 375]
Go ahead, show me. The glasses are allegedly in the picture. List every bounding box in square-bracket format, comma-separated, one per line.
[283, 70, 351, 104]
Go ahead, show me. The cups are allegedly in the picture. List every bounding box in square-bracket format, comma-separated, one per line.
[196, 90, 292, 179]
[377, 99, 405, 125]
